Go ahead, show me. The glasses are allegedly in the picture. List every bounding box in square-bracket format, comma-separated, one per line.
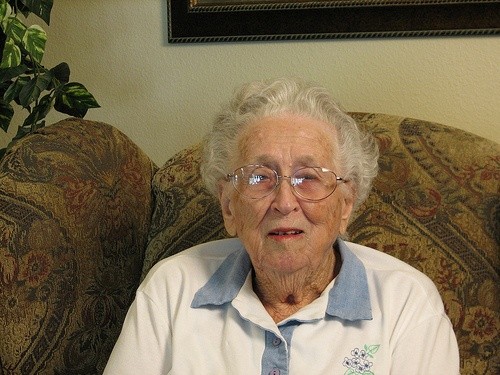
[225, 164, 350, 202]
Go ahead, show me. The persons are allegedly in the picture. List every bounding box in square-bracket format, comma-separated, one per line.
[103, 75, 460, 375]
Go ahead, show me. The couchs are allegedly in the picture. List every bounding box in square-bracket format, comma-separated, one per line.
[0, 112, 500, 375]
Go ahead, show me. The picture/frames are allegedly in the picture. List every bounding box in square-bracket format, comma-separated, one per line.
[167, 0, 500, 44]
